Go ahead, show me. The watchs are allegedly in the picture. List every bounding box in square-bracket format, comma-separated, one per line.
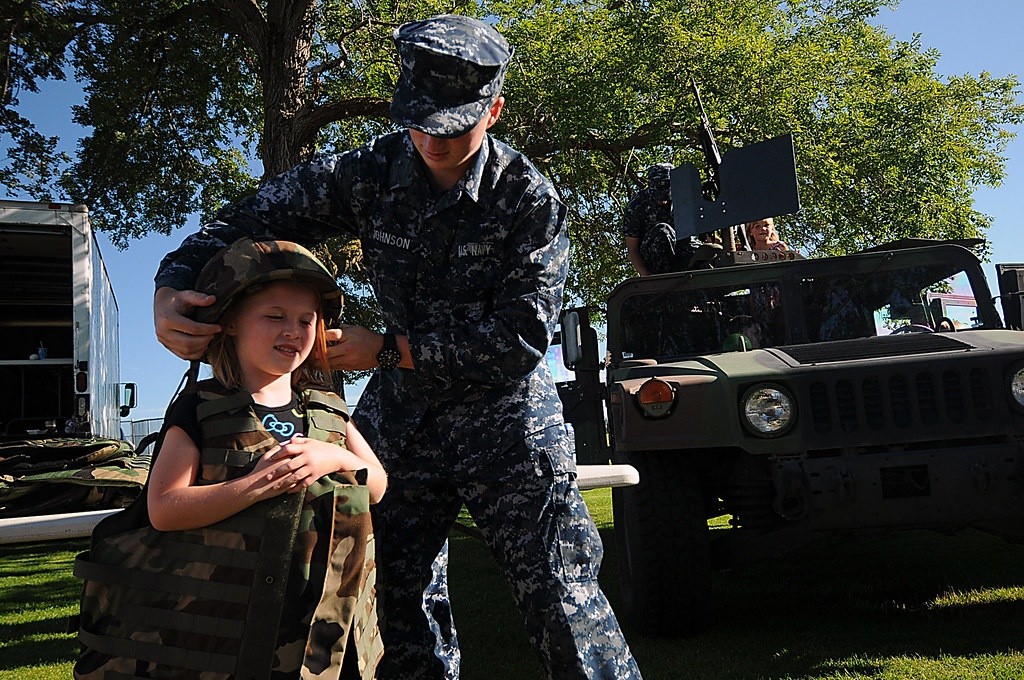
[377, 334, 401, 369]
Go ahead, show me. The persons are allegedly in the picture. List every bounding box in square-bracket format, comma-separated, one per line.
[154, 18, 641, 680]
[738, 218, 788, 250]
[623, 163, 705, 274]
[146, 234, 389, 533]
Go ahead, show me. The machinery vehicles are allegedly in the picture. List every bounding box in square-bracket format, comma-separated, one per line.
[557, 74, 1024, 589]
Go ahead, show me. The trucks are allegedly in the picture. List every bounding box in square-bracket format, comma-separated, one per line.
[1, 199, 138, 437]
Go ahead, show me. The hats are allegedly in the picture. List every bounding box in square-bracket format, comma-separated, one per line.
[647, 163, 675, 202]
[388, 14, 516, 140]
[190, 233, 344, 323]
